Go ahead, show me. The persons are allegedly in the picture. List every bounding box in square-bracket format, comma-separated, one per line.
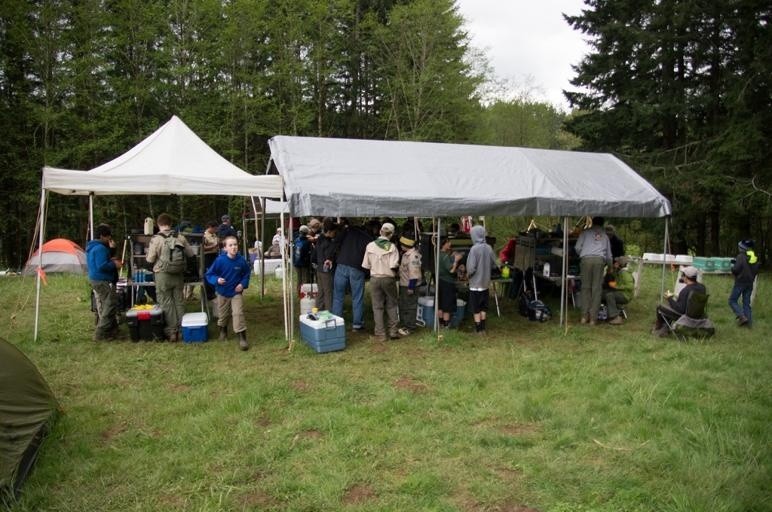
[728, 239, 760, 329]
[650, 265, 707, 336]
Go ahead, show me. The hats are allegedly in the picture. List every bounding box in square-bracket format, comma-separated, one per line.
[400, 233, 415, 247]
[738, 239, 755, 249]
[679, 265, 698, 278]
[299, 225, 311, 234]
[380, 222, 395, 233]
[605, 224, 616, 234]
[222, 215, 230, 219]
[614, 255, 627, 264]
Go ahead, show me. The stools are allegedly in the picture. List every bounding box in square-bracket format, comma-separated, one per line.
[614, 297, 631, 319]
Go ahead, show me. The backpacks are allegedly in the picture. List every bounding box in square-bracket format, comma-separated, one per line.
[294, 239, 307, 266]
[158, 231, 186, 273]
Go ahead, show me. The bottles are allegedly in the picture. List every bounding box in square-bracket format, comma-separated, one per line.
[542, 260, 551, 277]
[491, 261, 510, 280]
[598, 303, 607, 321]
[131, 263, 147, 282]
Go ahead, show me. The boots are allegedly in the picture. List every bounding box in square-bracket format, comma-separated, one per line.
[217, 325, 228, 341]
[238, 330, 249, 351]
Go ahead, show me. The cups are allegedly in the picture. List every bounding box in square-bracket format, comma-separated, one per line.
[312, 308, 318, 314]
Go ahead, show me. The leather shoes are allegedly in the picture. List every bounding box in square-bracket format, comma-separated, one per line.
[610, 314, 624, 324]
[736, 314, 748, 328]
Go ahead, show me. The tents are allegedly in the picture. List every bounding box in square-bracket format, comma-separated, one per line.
[17, 236, 92, 279]
[0, 336, 65, 511]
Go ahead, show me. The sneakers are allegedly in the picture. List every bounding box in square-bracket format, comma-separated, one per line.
[464, 327, 487, 337]
[92, 330, 125, 342]
[352, 324, 369, 332]
[580, 317, 597, 326]
[399, 326, 415, 335]
[439, 321, 452, 330]
[372, 333, 387, 344]
[170, 331, 182, 342]
[390, 328, 401, 339]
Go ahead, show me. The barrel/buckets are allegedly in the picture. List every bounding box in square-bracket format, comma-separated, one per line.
[143, 214, 154, 236]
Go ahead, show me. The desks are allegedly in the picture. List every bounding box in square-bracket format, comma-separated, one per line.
[456, 278, 517, 316]
[93, 280, 212, 323]
[530, 268, 583, 312]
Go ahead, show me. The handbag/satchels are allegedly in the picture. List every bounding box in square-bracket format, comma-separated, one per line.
[670, 314, 716, 338]
[520, 292, 552, 321]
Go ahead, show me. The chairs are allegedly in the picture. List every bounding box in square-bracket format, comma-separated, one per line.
[653, 291, 713, 339]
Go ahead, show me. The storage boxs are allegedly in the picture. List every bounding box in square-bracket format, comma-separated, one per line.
[299, 310, 346, 354]
[180, 312, 211, 344]
[126, 304, 167, 341]
[416, 296, 466, 327]
